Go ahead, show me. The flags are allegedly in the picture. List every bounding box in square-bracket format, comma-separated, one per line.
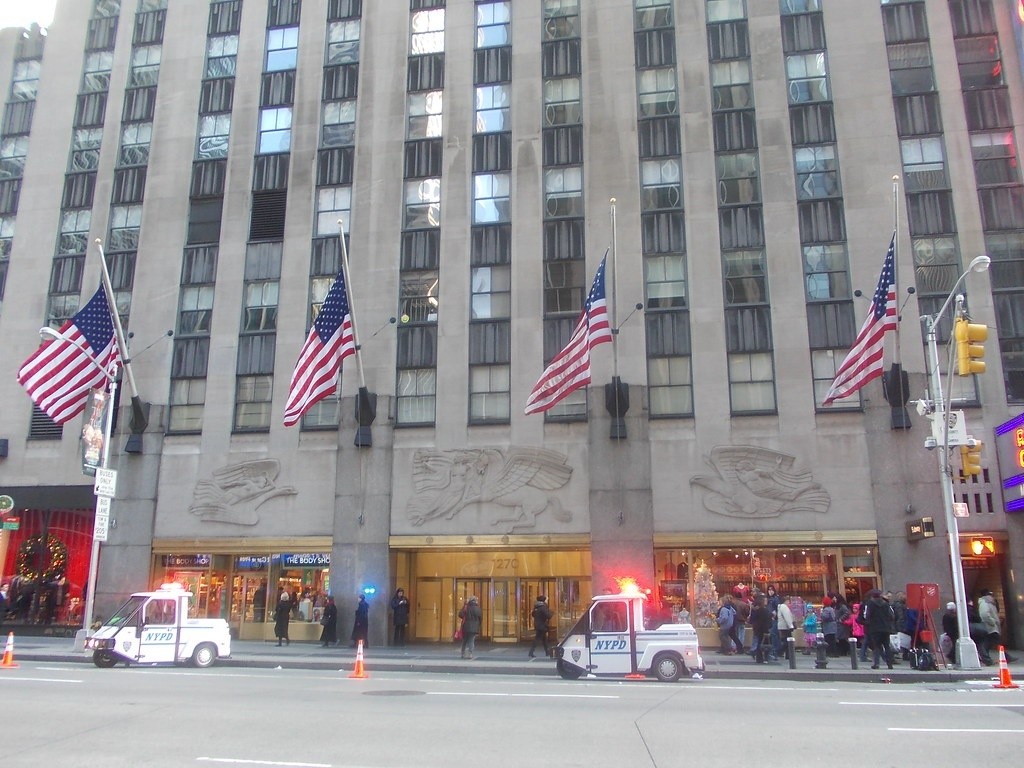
[822, 234, 898, 405]
[284, 265, 356, 426]
[523, 255, 612, 416]
[17, 282, 124, 426]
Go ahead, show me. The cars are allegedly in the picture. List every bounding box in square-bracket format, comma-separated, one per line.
[83, 589, 232, 668]
[551, 591, 707, 683]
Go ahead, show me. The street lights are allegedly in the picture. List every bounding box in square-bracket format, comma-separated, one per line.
[923, 255, 992, 671]
[39, 326, 118, 638]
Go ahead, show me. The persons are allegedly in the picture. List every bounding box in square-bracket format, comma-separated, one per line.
[252, 583, 266, 621]
[299, 589, 311, 619]
[820, 589, 904, 669]
[943, 590, 1019, 665]
[274, 592, 290, 647]
[351, 594, 370, 648]
[391, 588, 410, 645]
[716, 585, 795, 663]
[529, 595, 553, 657]
[320, 596, 338, 647]
[800, 605, 817, 655]
[459, 596, 483, 658]
[0, 583, 10, 601]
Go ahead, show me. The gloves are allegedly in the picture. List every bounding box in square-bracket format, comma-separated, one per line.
[790, 628, 794, 631]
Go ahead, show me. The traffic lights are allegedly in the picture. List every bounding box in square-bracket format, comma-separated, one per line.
[960, 438, 983, 477]
[955, 315, 987, 377]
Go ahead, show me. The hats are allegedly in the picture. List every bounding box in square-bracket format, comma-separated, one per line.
[823, 597, 832, 606]
[807, 603, 813, 609]
[853, 604, 861, 610]
[872, 589, 882, 597]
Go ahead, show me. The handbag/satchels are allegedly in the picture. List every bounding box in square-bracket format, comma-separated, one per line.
[887, 633, 901, 653]
[461, 605, 467, 626]
[855, 602, 872, 626]
[909, 648, 939, 672]
[321, 616, 331, 628]
[938, 633, 952, 655]
[897, 632, 911, 651]
[792, 622, 796, 629]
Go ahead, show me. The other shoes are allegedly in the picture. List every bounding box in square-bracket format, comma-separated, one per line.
[546, 651, 551, 656]
[529, 651, 536, 657]
[468, 653, 472, 658]
[718, 645, 1020, 670]
[462, 653, 465, 657]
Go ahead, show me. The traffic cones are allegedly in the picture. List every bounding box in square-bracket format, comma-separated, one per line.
[992, 645, 1019, 689]
[0, 630, 20, 668]
[346, 639, 370, 678]
